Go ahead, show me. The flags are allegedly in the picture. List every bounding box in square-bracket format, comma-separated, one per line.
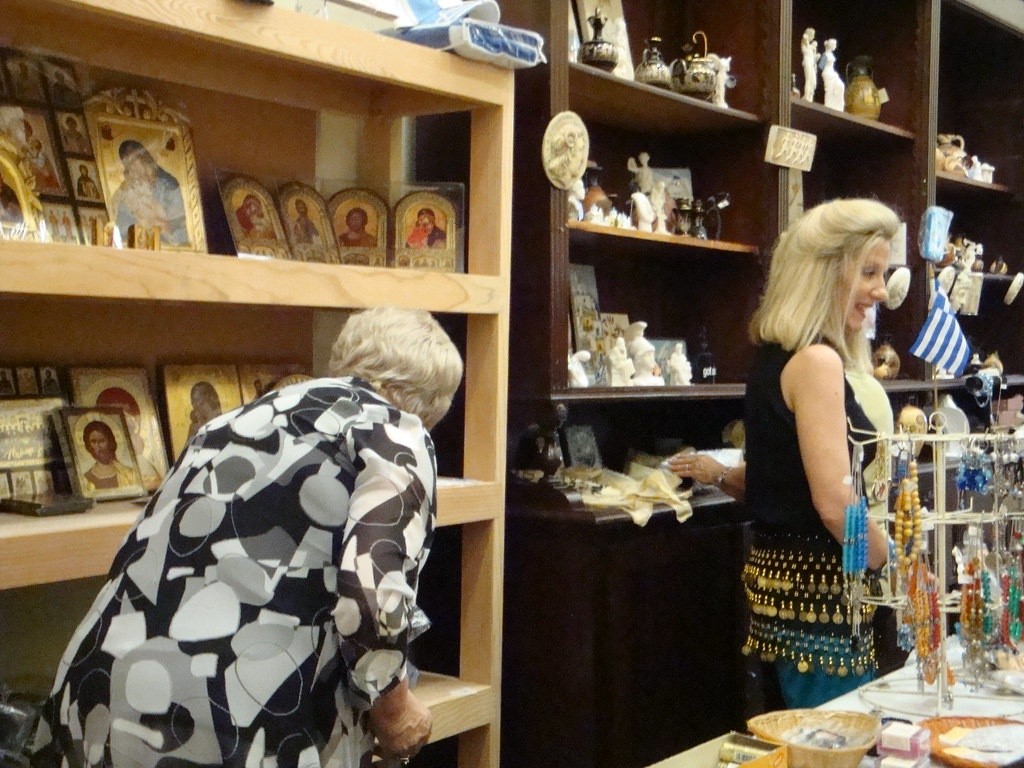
[909, 278, 969, 377]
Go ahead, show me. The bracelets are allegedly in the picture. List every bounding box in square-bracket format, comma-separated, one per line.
[714, 466, 730, 490]
[875, 557, 895, 580]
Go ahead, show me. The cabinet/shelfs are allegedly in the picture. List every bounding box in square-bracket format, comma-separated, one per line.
[0, 0, 1024, 768]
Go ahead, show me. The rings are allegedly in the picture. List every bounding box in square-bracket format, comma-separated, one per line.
[686, 464, 689, 470]
[400, 754, 410, 764]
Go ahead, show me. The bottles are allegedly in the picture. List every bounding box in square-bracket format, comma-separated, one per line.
[894, 396, 927, 458]
[872, 336, 900, 380]
[582, 40, 618, 72]
[844, 59, 880, 120]
[938, 134, 964, 173]
[635, 37, 671, 91]
[671, 52, 716, 98]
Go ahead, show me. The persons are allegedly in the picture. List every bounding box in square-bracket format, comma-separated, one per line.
[801, 27, 840, 109]
[32, 308, 465, 768]
[666, 316, 893, 675]
[636, 153, 655, 193]
[630, 337, 666, 386]
[666, 342, 692, 385]
[649, 181, 671, 234]
[740, 191, 938, 710]
[568, 179, 585, 221]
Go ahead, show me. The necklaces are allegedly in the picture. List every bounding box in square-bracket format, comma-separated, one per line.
[842, 443, 1024, 692]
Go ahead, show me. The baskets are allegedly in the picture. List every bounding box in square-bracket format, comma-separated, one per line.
[746, 710, 881, 768]
[917, 716, 1023, 768]
